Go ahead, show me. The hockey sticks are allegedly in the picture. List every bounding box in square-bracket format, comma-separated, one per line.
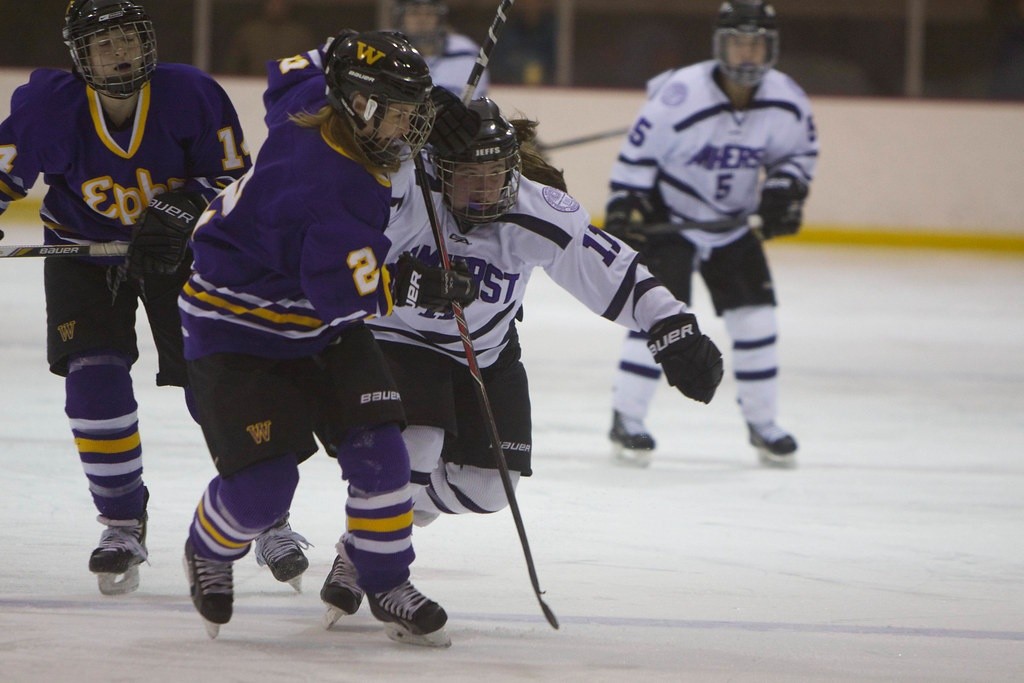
[597, 215, 747, 239]
[412, 145, 561, 634]
[458, 0, 514, 108]
[540, 120, 634, 154]
[0, 239, 130, 261]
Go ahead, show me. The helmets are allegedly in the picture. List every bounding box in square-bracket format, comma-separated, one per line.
[436, 98, 521, 226]
[325, 31, 436, 171]
[62, 1, 158, 99]
[711, 1, 782, 85]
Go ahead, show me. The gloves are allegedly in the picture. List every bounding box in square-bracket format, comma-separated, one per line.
[426, 86, 482, 159]
[602, 197, 651, 249]
[391, 253, 476, 311]
[645, 312, 724, 405]
[756, 176, 809, 239]
[127, 184, 214, 274]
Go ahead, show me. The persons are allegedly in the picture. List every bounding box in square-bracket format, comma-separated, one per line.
[178, 28, 452, 648]
[317, 1, 725, 628]
[604, 0, 820, 468]
[1, 0, 314, 594]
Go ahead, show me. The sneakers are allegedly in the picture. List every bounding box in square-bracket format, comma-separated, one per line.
[319, 551, 366, 631]
[609, 413, 657, 464]
[366, 581, 453, 649]
[747, 421, 798, 466]
[184, 540, 234, 641]
[90, 523, 151, 594]
[255, 522, 313, 594]
